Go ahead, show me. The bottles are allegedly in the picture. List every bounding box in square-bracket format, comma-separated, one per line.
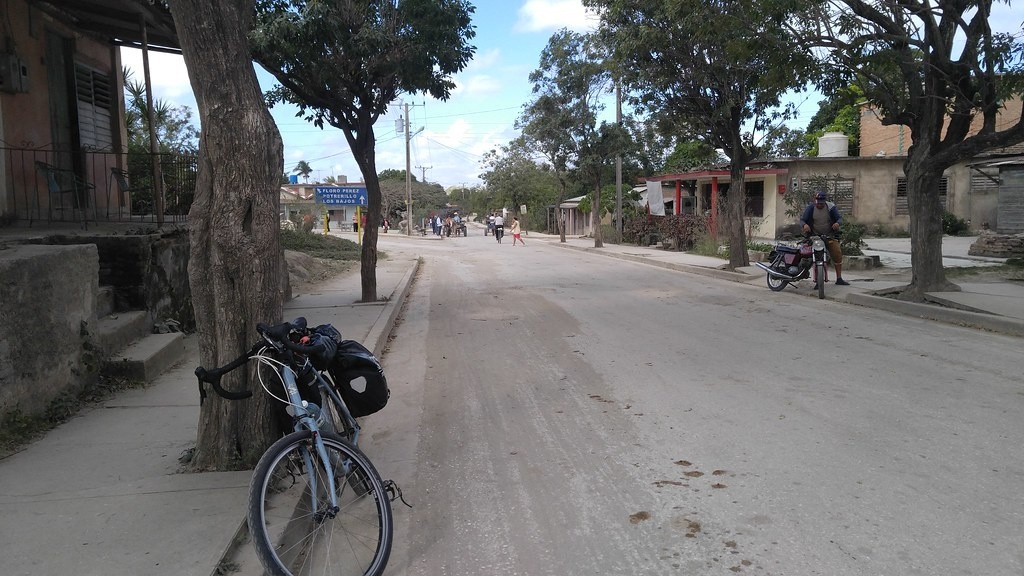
[302, 400, 332, 434]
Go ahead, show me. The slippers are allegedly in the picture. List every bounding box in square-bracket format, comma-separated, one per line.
[835, 281, 850, 285]
[814, 284, 818, 289]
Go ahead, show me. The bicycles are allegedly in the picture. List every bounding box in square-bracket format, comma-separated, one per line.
[495, 226, 504, 244]
[195, 317, 404, 575]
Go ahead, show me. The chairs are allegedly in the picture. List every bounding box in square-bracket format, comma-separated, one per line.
[104, 166, 155, 222]
[28, 161, 101, 229]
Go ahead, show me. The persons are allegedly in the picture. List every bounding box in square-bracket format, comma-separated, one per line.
[425, 210, 468, 237]
[509, 217, 526, 247]
[325, 209, 331, 232]
[382, 216, 392, 233]
[493, 214, 505, 240]
[799, 189, 853, 286]
[361, 213, 367, 232]
[351, 211, 360, 232]
[483, 214, 496, 236]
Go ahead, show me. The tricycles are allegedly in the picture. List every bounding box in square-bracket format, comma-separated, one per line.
[484, 219, 500, 236]
[446, 220, 467, 237]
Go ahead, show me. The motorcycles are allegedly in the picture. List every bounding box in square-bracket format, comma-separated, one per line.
[755, 228, 834, 300]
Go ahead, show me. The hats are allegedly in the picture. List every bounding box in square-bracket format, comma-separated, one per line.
[815, 192, 826, 204]
